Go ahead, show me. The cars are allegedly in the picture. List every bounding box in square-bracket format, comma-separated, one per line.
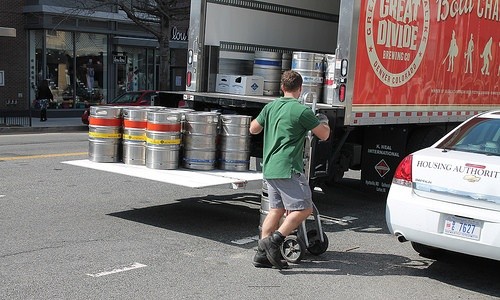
[81, 89, 157, 129]
[383, 111, 500, 274]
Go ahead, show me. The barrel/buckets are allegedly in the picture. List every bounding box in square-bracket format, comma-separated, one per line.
[146, 112, 183, 171]
[162, 108, 196, 161]
[253, 51, 282, 96]
[88, 106, 122, 163]
[290, 51, 325, 104]
[122, 109, 155, 165]
[278, 53, 292, 96]
[218, 114, 252, 172]
[323, 54, 336, 105]
[183, 112, 221, 170]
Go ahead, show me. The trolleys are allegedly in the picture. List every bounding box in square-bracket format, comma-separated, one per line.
[278, 116, 329, 264]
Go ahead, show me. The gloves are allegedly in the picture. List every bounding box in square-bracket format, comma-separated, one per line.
[314, 114, 329, 124]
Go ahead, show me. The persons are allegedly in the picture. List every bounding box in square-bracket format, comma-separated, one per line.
[38, 80, 53, 122]
[250, 70, 330, 270]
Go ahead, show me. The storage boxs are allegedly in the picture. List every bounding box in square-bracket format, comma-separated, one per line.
[230, 75, 264, 96]
[215, 74, 231, 94]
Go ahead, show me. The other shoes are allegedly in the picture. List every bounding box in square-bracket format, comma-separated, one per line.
[40, 119, 47, 122]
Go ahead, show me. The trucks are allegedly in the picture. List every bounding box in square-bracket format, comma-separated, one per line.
[176, 0, 500, 200]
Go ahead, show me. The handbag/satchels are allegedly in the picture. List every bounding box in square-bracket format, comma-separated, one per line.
[35, 101, 40, 108]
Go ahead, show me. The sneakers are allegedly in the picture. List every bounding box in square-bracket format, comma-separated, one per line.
[258, 235, 283, 270]
[253, 250, 287, 269]
